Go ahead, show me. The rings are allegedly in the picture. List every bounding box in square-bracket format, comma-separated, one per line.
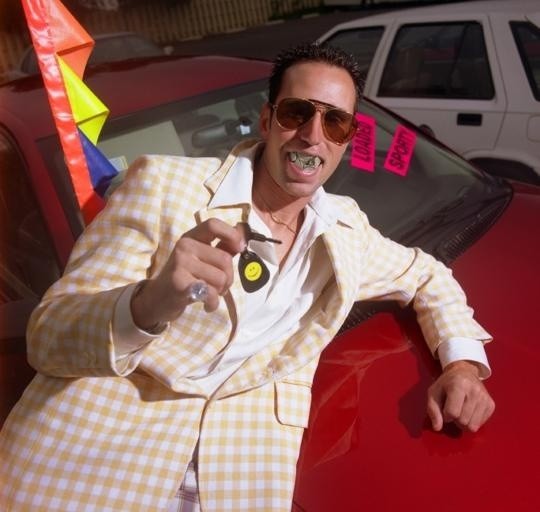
[190, 279, 208, 302]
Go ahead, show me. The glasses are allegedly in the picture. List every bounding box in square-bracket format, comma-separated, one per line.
[270, 96, 360, 148]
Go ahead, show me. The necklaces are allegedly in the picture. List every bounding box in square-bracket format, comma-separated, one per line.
[253, 186, 300, 236]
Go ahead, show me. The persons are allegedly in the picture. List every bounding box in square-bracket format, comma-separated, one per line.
[1, 38, 499, 512]
